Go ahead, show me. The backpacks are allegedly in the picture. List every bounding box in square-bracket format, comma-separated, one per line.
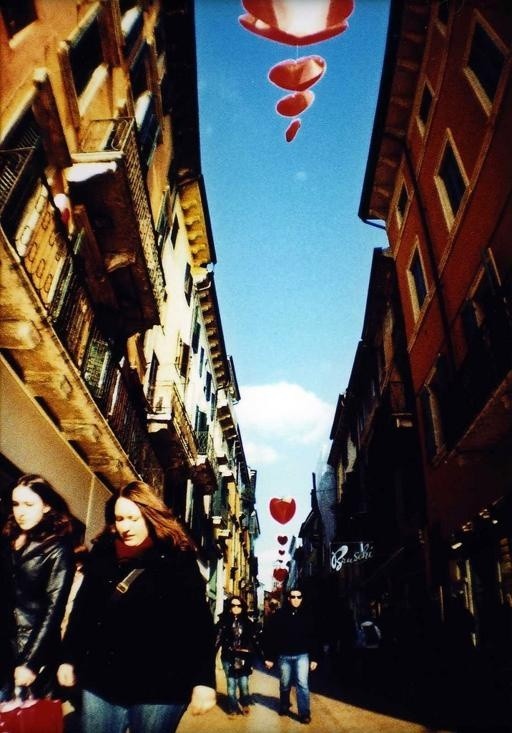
[362, 623, 380, 648]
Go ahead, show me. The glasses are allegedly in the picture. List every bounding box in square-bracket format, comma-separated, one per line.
[290, 596, 302, 599]
[231, 604, 242, 607]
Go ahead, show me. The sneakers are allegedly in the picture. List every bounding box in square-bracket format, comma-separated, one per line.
[280, 705, 289, 716]
[242, 705, 250, 716]
[227, 702, 237, 712]
[297, 711, 311, 723]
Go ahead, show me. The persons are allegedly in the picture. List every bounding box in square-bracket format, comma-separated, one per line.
[0, 473, 76, 707]
[214, 588, 320, 724]
[57, 480, 217, 733]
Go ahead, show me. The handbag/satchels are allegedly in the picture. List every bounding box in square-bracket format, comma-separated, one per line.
[0, 685, 65, 733]
[229, 650, 254, 677]
